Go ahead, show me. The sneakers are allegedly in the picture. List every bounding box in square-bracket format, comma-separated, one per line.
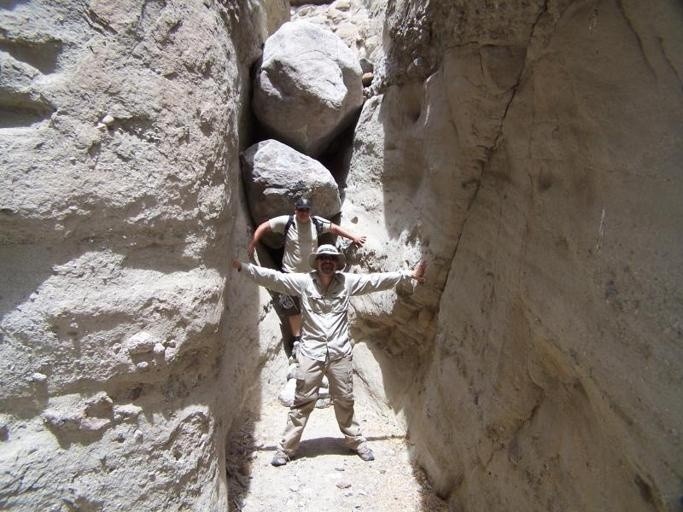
[353, 443, 374, 461]
[270, 451, 291, 465]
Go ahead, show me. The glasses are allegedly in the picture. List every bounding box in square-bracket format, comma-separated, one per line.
[317, 254, 338, 261]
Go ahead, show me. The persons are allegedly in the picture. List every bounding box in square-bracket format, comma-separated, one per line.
[247, 197, 366, 348]
[231, 243, 428, 466]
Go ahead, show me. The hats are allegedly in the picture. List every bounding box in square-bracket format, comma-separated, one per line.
[308, 243, 345, 270]
[294, 198, 311, 210]
